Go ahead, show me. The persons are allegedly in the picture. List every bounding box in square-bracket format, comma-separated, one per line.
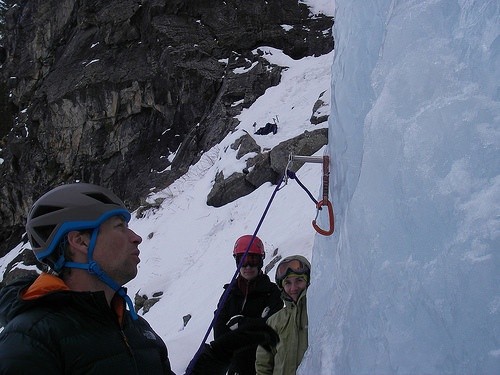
[213, 235, 285, 375]
[254, 255, 312, 375]
[0, 183, 176, 375]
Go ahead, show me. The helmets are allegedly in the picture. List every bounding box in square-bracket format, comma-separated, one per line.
[233, 235, 265, 259]
[25, 183, 131, 262]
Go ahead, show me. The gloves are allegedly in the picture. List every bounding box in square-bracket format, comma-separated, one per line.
[210, 321, 280, 362]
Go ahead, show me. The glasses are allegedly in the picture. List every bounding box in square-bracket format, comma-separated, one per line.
[277, 259, 310, 288]
[236, 256, 264, 267]
